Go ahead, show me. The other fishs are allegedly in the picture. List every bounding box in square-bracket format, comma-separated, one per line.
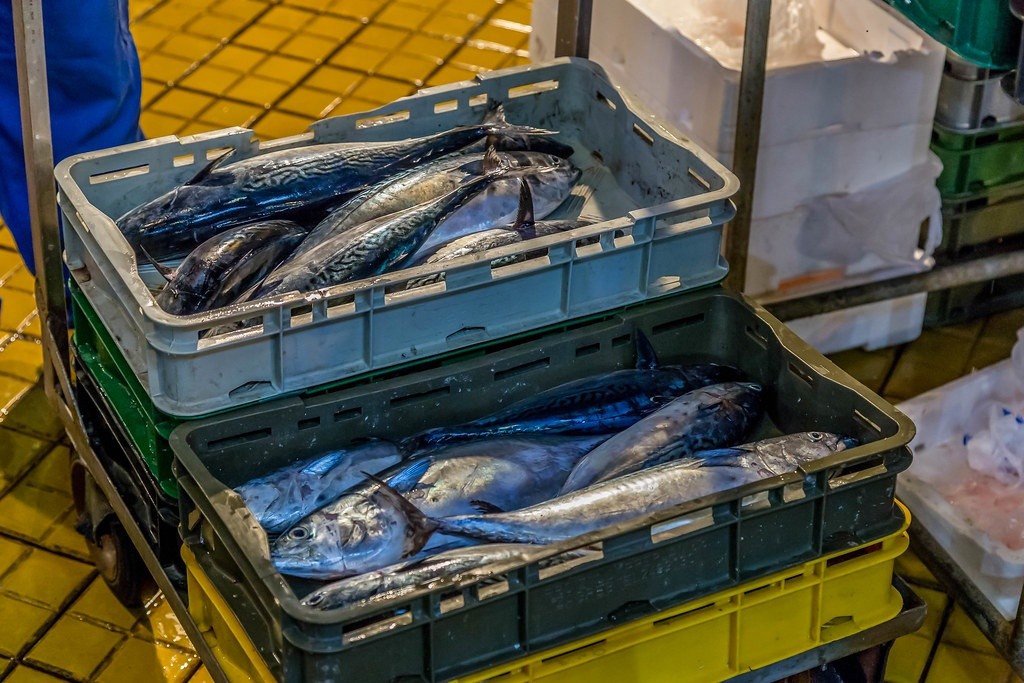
[110, 93, 862, 618]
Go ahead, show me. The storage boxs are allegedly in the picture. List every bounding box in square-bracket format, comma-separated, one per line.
[53, 0, 1024, 683]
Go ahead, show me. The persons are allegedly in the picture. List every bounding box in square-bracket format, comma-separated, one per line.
[0, 0, 142, 380]
